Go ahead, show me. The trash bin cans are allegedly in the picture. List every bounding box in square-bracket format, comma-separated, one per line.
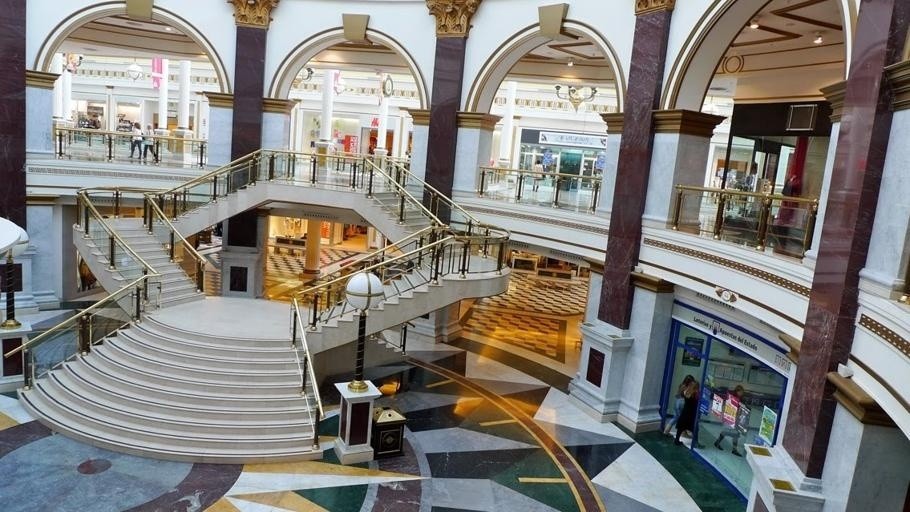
[371, 405, 408, 460]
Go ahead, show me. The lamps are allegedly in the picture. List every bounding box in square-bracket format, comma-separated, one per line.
[812, 31, 823, 44]
[555, 85, 597, 112]
[567, 57, 573, 68]
[750, 16, 759, 29]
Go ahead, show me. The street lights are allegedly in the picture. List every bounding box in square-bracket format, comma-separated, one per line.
[345, 263, 386, 392]
[1, 213, 33, 331]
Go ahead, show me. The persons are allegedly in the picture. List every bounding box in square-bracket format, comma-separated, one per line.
[92, 115, 101, 130]
[663, 375, 694, 438]
[143, 125, 158, 163]
[533, 160, 543, 192]
[674, 381, 705, 449]
[129, 123, 141, 160]
[714, 385, 744, 457]
[782, 174, 802, 224]
